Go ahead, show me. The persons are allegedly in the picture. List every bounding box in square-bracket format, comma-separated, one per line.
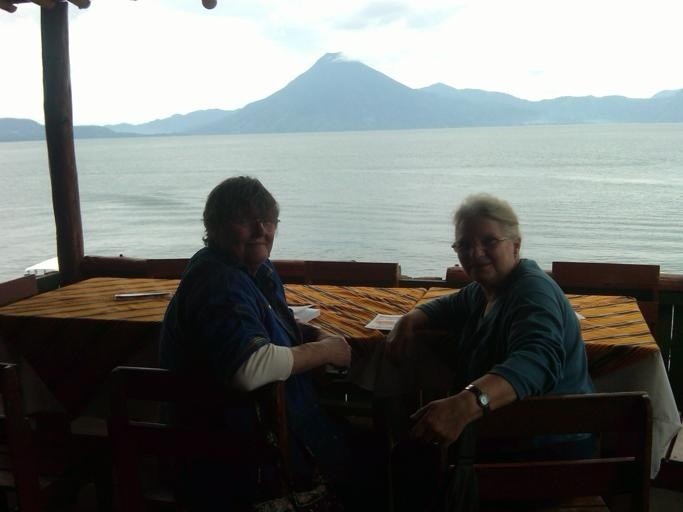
[158, 176, 353, 508]
[381, 191, 605, 511]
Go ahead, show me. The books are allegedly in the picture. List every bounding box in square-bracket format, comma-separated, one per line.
[110, 290, 173, 302]
[363, 313, 405, 331]
[286, 302, 321, 323]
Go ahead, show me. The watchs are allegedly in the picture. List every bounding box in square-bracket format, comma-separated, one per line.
[463, 383, 490, 419]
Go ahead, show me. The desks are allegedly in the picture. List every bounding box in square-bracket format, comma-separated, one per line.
[1, 277, 671, 438]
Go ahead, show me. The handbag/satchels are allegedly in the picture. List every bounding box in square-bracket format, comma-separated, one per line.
[252, 470, 346, 512]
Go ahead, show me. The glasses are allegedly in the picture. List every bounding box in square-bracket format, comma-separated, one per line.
[451, 237, 510, 253]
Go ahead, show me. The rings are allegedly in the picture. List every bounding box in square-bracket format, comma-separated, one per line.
[430, 438, 440, 447]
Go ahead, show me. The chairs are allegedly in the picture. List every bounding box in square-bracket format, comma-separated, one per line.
[550, 263, 659, 349]
[106, 364, 328, 508]
[439, 390, 654, 510]
